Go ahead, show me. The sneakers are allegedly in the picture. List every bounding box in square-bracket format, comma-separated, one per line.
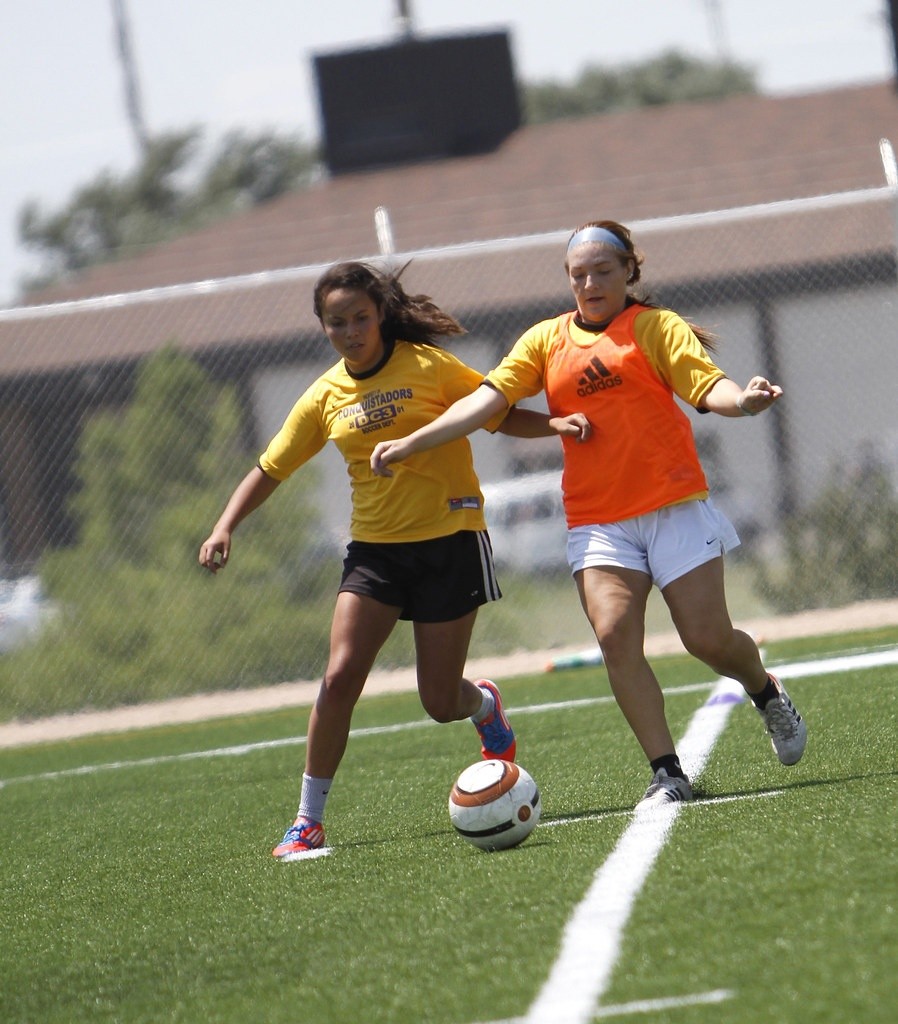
[749, 671, 807, 765]
[469, 679, 516, 763]
[632, 767, 693, 815]
[272, 816, 325, 857]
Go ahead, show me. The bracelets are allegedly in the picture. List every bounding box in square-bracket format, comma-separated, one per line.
[735, 397, 758, 416]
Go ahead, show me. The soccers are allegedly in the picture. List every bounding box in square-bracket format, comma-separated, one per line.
[448, 759, 541, 853]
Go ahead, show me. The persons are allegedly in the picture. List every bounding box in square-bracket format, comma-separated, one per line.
[370, 221, 807, 813]
[200, 261, 591, 859]
